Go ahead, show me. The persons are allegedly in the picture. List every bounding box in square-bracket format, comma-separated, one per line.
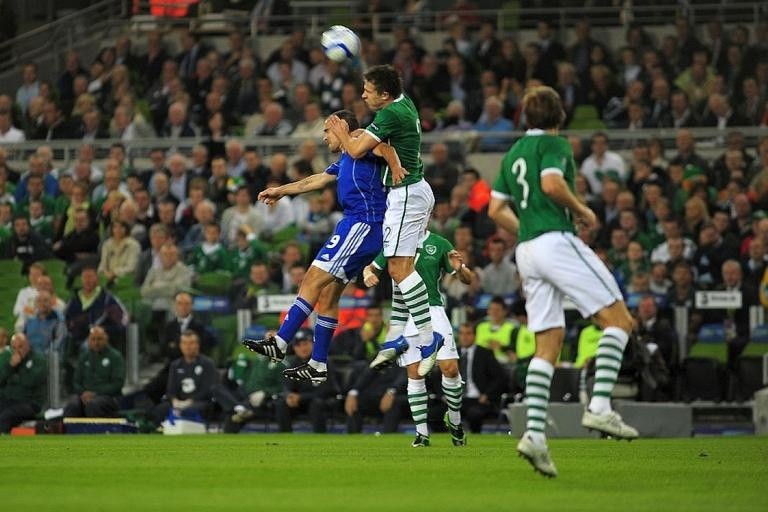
[363, 227, 472, 445]
[328, 66, 444, 375]
[487, 86, 637, 479]
[0, 0, 767, 433]
[242, 111, 409, 385]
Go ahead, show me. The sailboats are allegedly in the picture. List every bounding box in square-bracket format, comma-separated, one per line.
[322, 25, 360, 64]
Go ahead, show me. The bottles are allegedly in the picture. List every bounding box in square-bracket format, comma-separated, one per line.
[291, 328, 313, 345]
[226, 177, 245, 192]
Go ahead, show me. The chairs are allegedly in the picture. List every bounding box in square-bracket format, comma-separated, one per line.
[580, 406, 639, 442]
[443, 412, 466, 446]
[412, 436, 430, 447]
[416, 332, 444, 377]
[240, 337, 285, 364]
[369, 336, 408, 371]
[516, 430, 558, 477]
[282, 362, 328, 383]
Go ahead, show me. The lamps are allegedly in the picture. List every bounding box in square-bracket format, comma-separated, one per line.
[0, 258, 235, 420]
[684, 304, 767, 410]
